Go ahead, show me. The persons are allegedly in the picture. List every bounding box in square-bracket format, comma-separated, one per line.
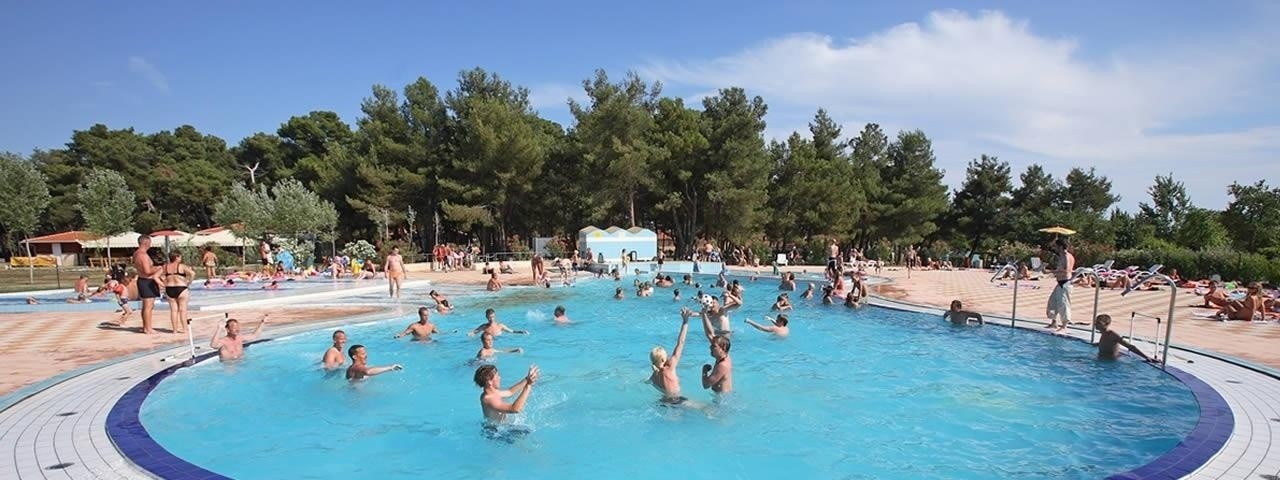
[210, 313, 269, 361]
[635, 239, 869, 336]
[651, 306, 731, 391]
[434, 300, 451, 312]
[430, 290, 446, 304]
[394, 307, 458, 340]
[1044, 239, 1075, 332]
[433, 233, 513, 290]
[466, 308, 530, 338]
[133, 235, 163, 333]
[323, 331, 346, 368]
[531, 247, 640, 321]
[473, 364, 542, 422]
[346, 344, 403, 380]
[384, 245, 407, 298]
[152, 250, 196, 334]
[478, 332, 523, 359]
[1084, 267, 1267, 362]
[874, 244, 1029, 280]
[26, 256, 133, 328]
[943, 300, 984, 326]
[203, 239, 375, 289]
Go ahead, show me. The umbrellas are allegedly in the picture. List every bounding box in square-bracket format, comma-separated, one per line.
[1038, 226, 1077, 237]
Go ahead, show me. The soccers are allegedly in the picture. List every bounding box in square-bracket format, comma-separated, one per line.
[699, 294, 713, 310]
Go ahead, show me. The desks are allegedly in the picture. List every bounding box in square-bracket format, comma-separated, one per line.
[88, 256, 135, 268]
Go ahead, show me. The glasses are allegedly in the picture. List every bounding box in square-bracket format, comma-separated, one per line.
[1247, 285, 1257, 290]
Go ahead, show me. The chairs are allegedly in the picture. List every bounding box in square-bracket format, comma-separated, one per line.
[1131, 264, 1166, 285]
[1099, 265, 1140, 289]
[1070, 259, 1116, 285]
[776, 253, 788, 266]
[1031, 256, 1049, 272]
